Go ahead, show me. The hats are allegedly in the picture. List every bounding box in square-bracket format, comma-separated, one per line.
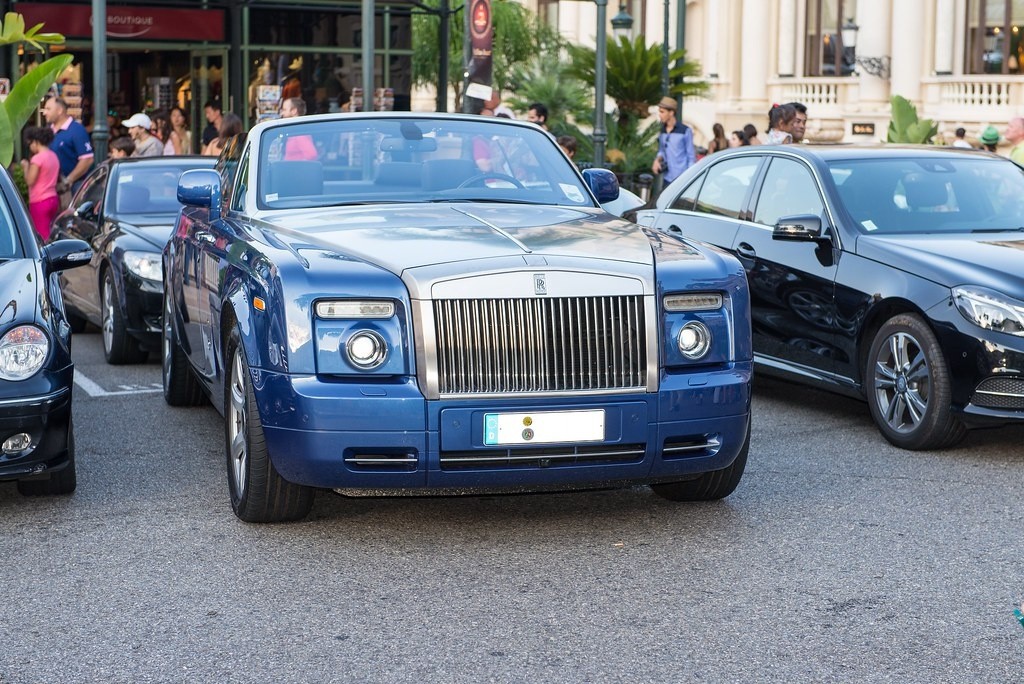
[494, 106, 514, 120]
[979, 126, 1001, 145]
[121, 113, 151, 130]
[658, 96, 678, 112]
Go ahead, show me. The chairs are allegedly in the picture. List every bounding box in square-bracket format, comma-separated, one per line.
[269, 159, 324, 198]
[422, 157, 486, 192]
[117, 187, 152, 211]
[372, 161, 422, 192]
[900, 172, 948, 212]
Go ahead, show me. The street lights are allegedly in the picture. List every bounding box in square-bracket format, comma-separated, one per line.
[593, 0, 635, 183]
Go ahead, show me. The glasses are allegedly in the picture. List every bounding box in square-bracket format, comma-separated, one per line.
[27, 140, 33, 149]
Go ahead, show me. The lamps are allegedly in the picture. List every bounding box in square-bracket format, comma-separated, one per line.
[840, 16, 890, 79]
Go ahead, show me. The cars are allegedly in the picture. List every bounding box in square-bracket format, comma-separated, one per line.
[158, 108, 755, 527]
[624, 144, 1021, 451]
[45, 154, 236, 376]
[0, 165, 95, 513]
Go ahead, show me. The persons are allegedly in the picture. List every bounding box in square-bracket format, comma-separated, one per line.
[976, 124, 1002, 152]
[1004, 117, 1024, 165]
[200, 100, 224, 155]
[765, 102, 808, 145]
[695, 145, 708, 160]
[204, 111, 241, 156]
[952, 128, 973, 148]
[652, 96, 696, 188]
[708, 123, 729, 154]
[20, 126, 60, 239]
[106, 106, 193, 186]
[730, 130, 750, 147]
[42, 97, 96, 211]
[280, 97, 317, 161]
[473, 89, 576, 184]
[744, 124, 761, 144]
